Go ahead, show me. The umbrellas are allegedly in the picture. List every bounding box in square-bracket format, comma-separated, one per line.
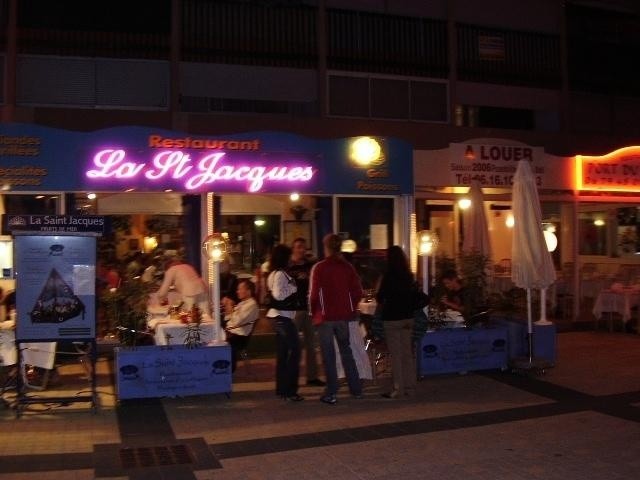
[462, 179, 494, 262]
[508, 159, 556, 376]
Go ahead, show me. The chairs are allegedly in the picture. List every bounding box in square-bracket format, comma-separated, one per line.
[367, 341, 394, 387]
[224, 319, 260, 369]
[532, 261, 640, 333]
[467, 308, 493, 331]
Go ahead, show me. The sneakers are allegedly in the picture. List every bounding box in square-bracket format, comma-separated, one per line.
[403, 386, 418, 402]
[320, 393, 337, 404]
[380, 387, 402, 400]
[305, 377, 329, 386]
[352, 391, 364, 399]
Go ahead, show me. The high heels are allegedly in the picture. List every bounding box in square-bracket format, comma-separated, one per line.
[279, 391, 305, 402]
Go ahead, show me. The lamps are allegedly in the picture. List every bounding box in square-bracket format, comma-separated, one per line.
[415, 229, 440, 256]
[203, 232, 231, 264]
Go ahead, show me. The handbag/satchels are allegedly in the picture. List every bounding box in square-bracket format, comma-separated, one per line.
[411, 287, 432, 312]
[267, 277, 311, 311]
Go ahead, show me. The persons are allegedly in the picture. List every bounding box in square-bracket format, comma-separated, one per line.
[266, 244, 305, 401]
[308, 234, 363, 405]
[285, 238, 326, 386]
[95, 252, 164, 335]
[158, 259, 210, 319]
[440, 269, 471, 316]
[222, 279, 260, 373]
[0, 286, 59, 386]
[374, 246, 419, 399]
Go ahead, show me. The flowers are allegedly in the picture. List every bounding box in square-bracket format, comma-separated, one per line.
[178, 303, 204, 328]
